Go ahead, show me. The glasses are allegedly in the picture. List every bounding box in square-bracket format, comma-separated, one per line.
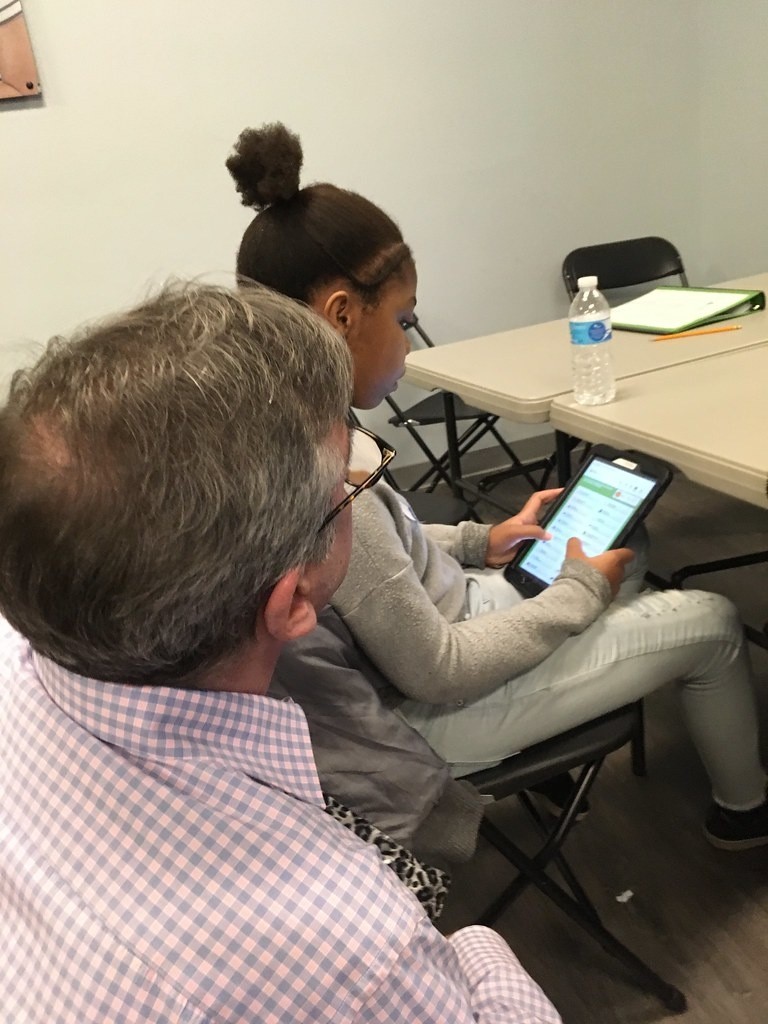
[320, 423, 397, 538]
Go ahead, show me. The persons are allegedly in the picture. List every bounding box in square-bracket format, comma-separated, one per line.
[0, 287, 562, 1024]
[225, 124, 768, 850]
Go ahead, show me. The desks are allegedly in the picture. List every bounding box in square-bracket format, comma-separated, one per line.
[547, 342, 768, 650]
[402, 268, 768, 486]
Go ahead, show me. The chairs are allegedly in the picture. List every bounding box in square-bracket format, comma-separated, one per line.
[315, 238, 690, 1013]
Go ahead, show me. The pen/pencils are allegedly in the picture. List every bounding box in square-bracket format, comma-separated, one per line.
[649, 325, 742, 342]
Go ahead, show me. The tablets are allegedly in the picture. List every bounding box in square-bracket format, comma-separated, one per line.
[504, 443, 673, 599]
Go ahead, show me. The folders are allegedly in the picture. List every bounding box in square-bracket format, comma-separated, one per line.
[610, 284, 767, 335]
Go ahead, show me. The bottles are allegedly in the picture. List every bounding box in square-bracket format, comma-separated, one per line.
[568, 276, 615, 405]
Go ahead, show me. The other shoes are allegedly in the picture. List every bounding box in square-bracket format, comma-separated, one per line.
[702, 803, 768, 850]
[531, 771, 590, 823]
